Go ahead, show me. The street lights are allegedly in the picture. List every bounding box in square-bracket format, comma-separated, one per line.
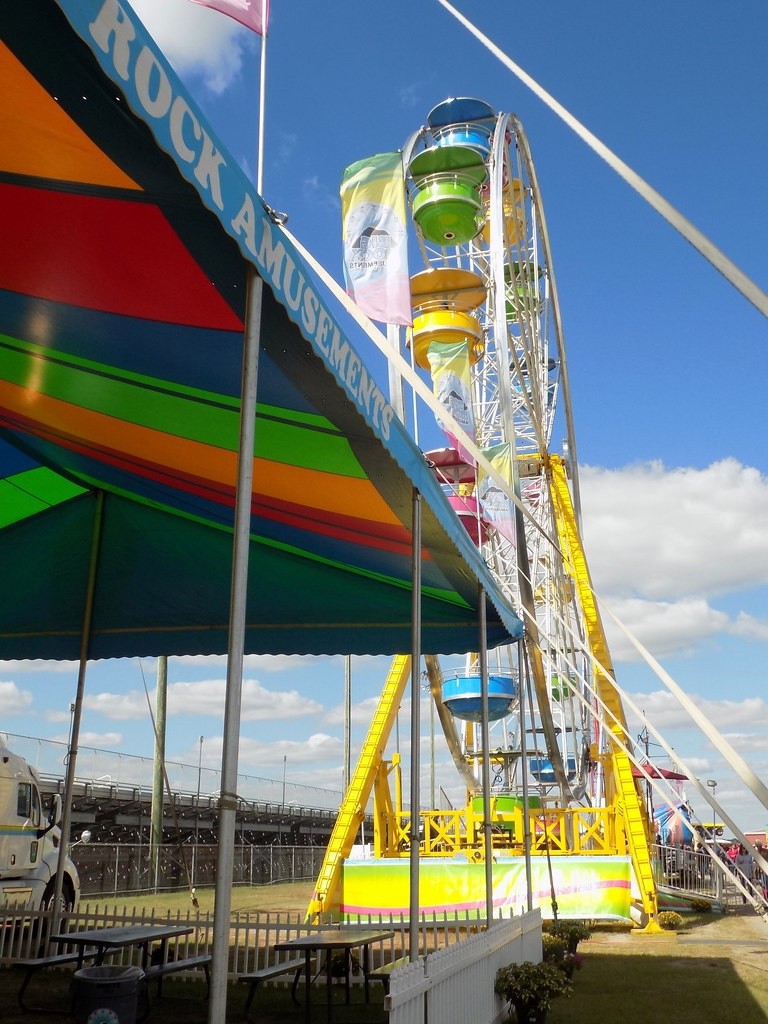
[707, 779, 717, 859]
[193, 735, 204, 884]
[278, 755, 287, 879]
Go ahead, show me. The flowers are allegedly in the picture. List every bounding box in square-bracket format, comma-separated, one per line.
[550, 950, 584, 971]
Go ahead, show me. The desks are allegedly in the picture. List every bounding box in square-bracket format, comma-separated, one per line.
[273, 929, 396, 1024]
[48, 925, 194, 1024]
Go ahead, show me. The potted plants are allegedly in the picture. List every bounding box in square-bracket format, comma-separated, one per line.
[688, 898, 713, 914]
[658, 911, 683, 931]
[493, 959, 579, 1024]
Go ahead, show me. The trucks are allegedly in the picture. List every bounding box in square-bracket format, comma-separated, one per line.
[0, 738, 92, 939]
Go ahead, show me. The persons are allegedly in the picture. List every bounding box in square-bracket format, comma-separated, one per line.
[657, 834, 768, 911]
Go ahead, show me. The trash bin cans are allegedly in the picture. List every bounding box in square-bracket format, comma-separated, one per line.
[71, 965, 145, 1024]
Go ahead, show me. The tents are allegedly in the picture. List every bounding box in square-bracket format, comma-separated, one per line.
[0, 0, 523, 1024]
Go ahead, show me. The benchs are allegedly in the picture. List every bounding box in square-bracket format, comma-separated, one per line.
[9, 946, 427, 1024]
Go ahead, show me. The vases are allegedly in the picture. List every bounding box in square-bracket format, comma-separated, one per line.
[563, 966, 574, 978]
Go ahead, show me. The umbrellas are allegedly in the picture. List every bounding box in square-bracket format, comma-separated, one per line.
[632, 763, 689, 830]
